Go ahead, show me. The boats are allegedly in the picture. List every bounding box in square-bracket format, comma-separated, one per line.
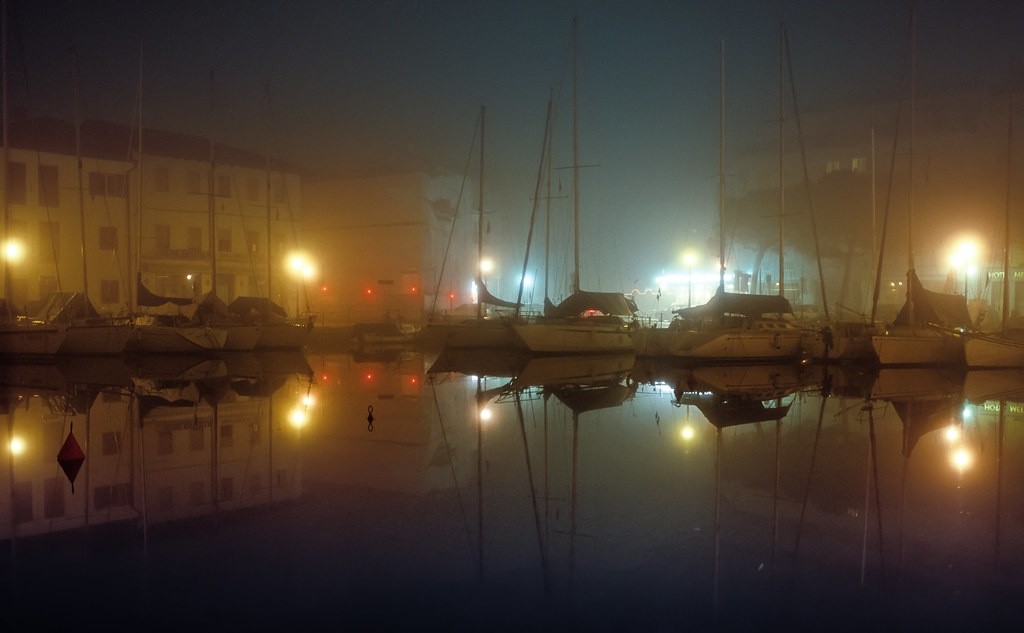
[349, 322, 418, 358]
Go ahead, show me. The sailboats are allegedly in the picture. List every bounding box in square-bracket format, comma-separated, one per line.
[510, 15, 645, 357]
[792, 8, 1024, 366]
[0, 68, 320, 357]
[650, 16, 808, 364]
[415, 104, 522, 348]
[417, 350, 672, 603]
[0, 354, 317, 545]
[676, 365, 1017, 618]
